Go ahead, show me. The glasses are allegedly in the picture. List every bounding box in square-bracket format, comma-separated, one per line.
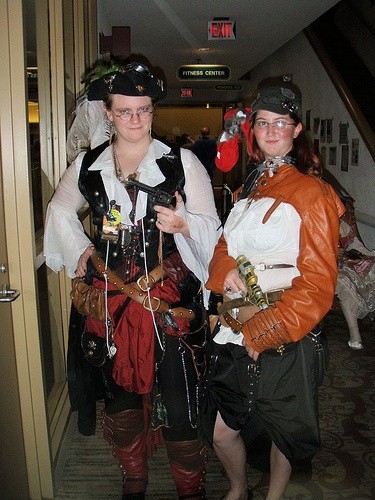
[255, 121, 295, 129]
[107, 105, 156, 121]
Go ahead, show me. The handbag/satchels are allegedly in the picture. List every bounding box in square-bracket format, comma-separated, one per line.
[78, 331, 108, 367]
[70, 277, 104, 321]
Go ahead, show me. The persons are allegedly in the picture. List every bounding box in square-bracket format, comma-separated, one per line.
[208, 85, 341, 500]
[179, 126, 216, 176]
[46, 66, 223, 500]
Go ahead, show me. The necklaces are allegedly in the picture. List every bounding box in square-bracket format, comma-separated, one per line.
[114, 148, 138, 185]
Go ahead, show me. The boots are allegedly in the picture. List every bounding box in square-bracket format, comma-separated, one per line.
[101, 409, 149, 500]
[164, 440, 208, 500]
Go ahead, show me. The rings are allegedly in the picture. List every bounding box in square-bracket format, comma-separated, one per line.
[175, 200, 183, 203]
[226, 287, 231, 291]
[246, 342, 250, 347]
[158, 219, 162, 224]
[243, 338, 247, 342]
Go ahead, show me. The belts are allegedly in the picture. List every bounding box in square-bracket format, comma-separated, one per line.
[91, 249, 194, 320]
[217, 287, 294, 334]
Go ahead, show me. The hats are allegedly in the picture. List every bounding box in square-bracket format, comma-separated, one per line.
[250, 86, 302, 121]
[81, 54, 168, 99]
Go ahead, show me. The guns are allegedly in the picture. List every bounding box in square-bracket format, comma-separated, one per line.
[124, 178, 174, 211]
[235, 257, 288, 354]
